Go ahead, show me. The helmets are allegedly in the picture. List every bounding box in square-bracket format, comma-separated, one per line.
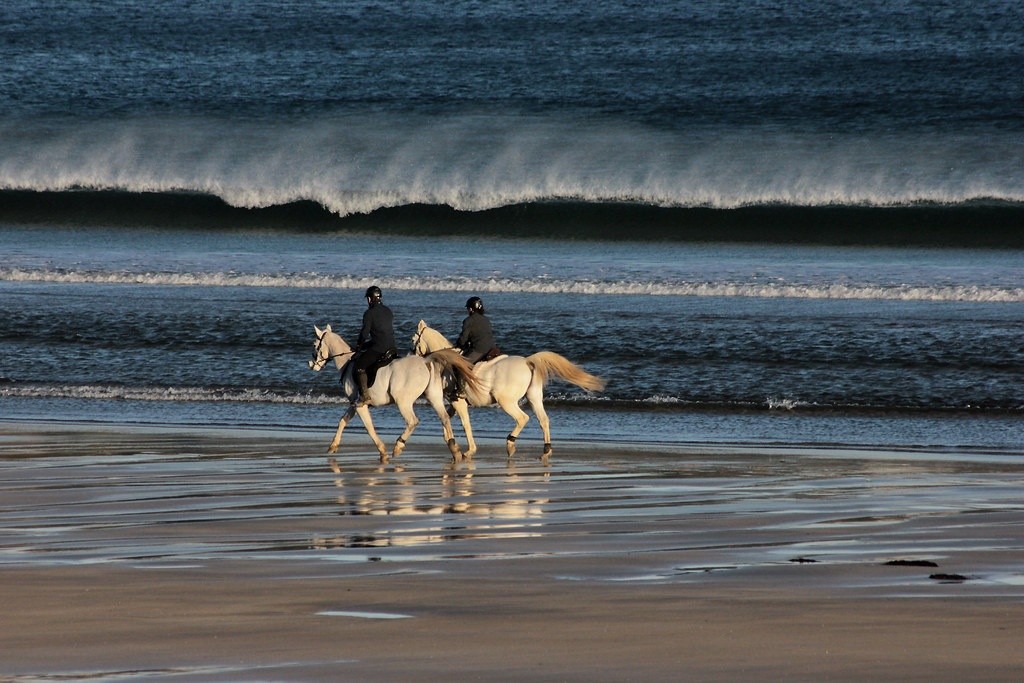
[465, 297, 483, 309]
[364, 286, 382, 298]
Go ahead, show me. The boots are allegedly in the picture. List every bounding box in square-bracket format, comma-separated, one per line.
[451, 372, 466, 394]
[352, 370, 371, 403]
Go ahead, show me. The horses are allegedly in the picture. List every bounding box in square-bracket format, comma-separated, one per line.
[307, 323, 488, 465]
[411, 319, 607, 462]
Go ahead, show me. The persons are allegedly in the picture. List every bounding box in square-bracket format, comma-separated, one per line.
[449, 297, 496, 399]
[350, 286, 397, 407]
